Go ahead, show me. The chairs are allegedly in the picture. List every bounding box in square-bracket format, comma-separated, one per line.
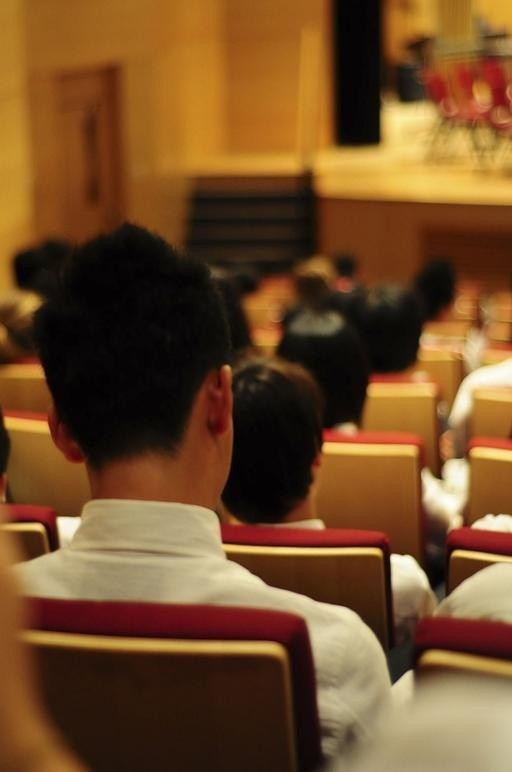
[0, 276, 512, 772]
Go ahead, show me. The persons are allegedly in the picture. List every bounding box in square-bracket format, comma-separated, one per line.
[3, 217, 401, 772]
[5, 240, 76, 364]
[222, 358, 441, 621]
[206, 249, 490, 587]
[439, 358, 512, 464]
